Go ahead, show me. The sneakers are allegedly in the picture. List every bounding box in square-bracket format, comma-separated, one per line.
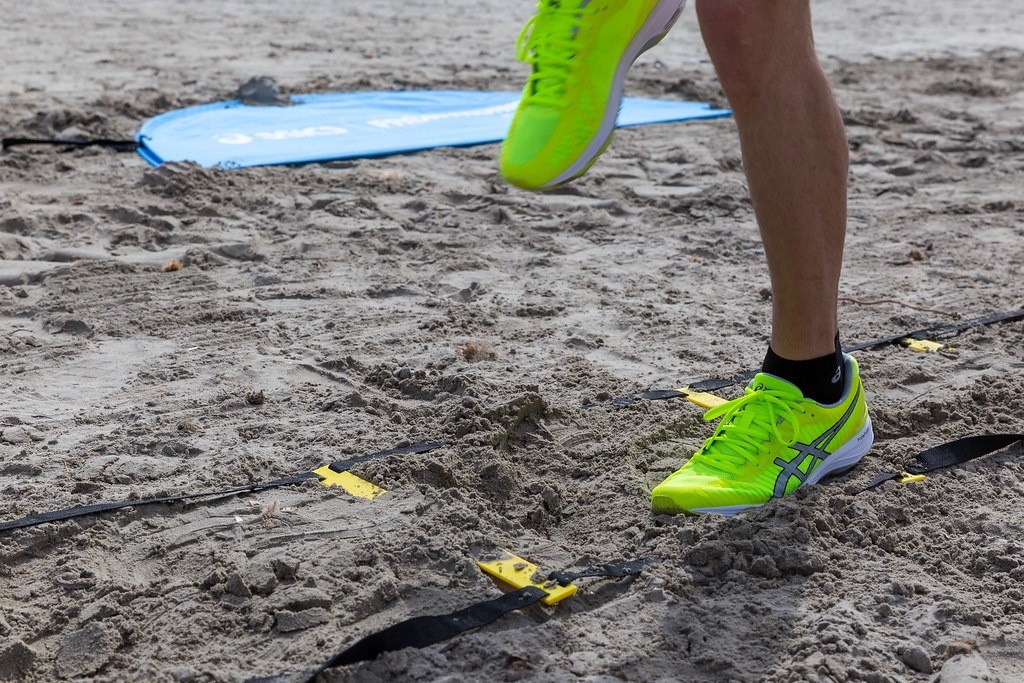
[499, 0, 686, 191]
[652, 352, 874, 517]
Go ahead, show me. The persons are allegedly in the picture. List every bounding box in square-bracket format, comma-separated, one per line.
[497, 0, 877, 520]
